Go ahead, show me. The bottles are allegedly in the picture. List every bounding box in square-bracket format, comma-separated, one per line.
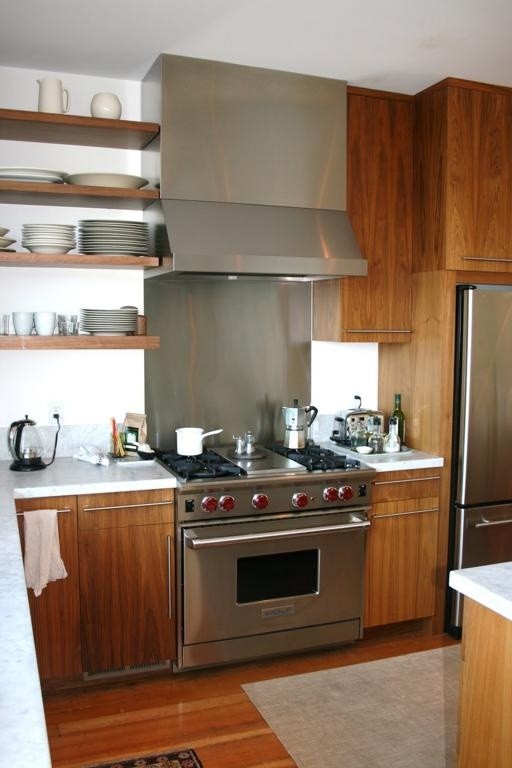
[392, 394, 406, 447]
[351, 423, 368, 452]
[383, 417, 400, 453]
[368, 417, 384, 454]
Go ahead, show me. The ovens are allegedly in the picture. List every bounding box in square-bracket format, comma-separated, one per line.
[177, 505, 372, 673]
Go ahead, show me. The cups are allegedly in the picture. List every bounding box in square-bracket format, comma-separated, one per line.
[37, 77, 70, 114]
[34, 311, 56, 334]
[2, 314, 15, 334]
[62, 322, 79, 335]
[57, 314, 76, 332]
[12, 311, 31, 333]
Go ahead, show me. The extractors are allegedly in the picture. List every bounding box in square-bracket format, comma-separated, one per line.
[144, 55, 368, 282]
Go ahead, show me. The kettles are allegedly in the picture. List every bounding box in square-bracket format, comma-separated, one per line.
[7, 414, 59, 471]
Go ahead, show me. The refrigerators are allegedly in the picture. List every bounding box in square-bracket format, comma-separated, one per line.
[443, 284, 512, 637]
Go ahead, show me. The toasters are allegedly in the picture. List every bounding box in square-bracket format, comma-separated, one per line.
[330, 396, 383, 443]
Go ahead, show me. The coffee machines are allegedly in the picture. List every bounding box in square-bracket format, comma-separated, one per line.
[274, 398, 317, 449]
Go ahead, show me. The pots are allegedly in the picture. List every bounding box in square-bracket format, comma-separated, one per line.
[176, 428, 222, 456]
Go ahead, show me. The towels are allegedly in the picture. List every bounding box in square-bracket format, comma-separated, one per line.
[23, 508, 68, 598]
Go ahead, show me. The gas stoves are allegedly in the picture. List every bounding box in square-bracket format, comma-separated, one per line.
[155, 440, 376, 522]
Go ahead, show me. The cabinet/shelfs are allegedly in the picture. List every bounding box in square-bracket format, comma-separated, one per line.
[344, 85, 412, 343]
[12, 494, 82, 695]
[77, 489, 177, 698]
[364, 467, 442, 637]
[412, 77, 512, 272]
[448, 561, 512, 768]
[0, 109, 160, 350]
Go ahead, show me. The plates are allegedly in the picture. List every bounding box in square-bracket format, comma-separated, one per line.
[330, 442, 414, 458]
[22, 224, 75, 253]
[83, 308, 140, 335]
[67, 173, 150, 189]
[77, 220, 151, 253]
[0, 166, 61, 183]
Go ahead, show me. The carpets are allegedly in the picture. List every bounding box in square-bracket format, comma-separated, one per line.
[96, 748, 205, 768]
[239, 642, 461, 768]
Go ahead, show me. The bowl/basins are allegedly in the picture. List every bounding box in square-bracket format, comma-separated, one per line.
[357, 446, 372, 453]
[137, 447, 159, 460]
